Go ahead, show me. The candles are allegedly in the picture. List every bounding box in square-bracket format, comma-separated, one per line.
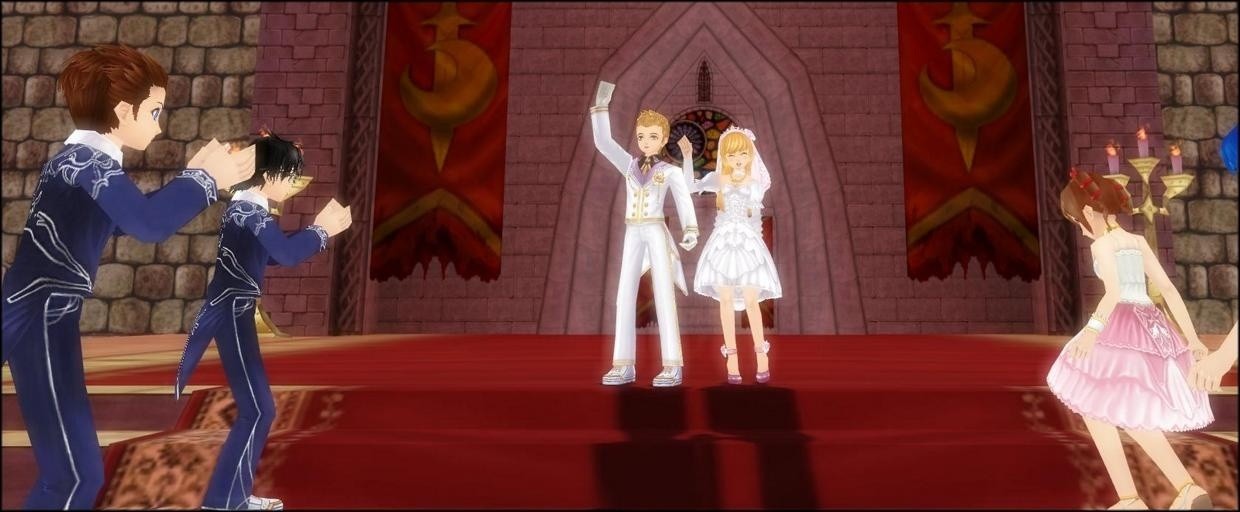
[1106, 133, 1184, 179]
